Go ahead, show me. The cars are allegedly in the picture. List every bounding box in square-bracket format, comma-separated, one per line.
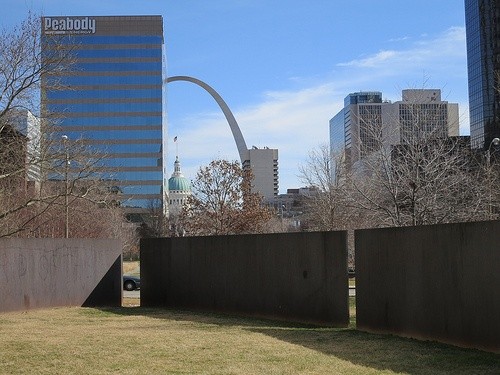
[122, 275, 140, 291]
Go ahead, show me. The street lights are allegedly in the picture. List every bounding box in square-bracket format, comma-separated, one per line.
[62, 135, 70, 238]
